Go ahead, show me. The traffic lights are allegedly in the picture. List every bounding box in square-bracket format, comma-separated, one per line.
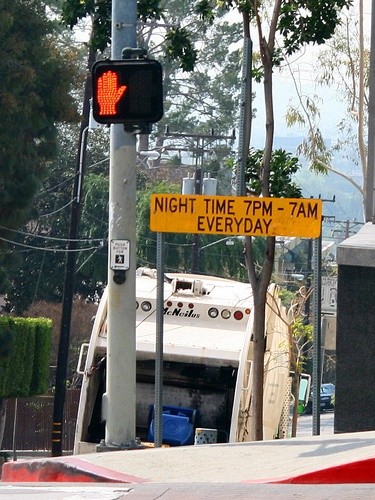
[91, 47, 161, 134]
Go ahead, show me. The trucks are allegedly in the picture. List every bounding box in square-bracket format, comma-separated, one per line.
[73, 267, 292, 454]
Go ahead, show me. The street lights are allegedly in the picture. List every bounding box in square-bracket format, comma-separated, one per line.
[52, 151, 160, 456]
[164, 131, 235, 273]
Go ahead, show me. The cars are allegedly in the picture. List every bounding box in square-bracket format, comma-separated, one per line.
[309, 383, 334, 413]
